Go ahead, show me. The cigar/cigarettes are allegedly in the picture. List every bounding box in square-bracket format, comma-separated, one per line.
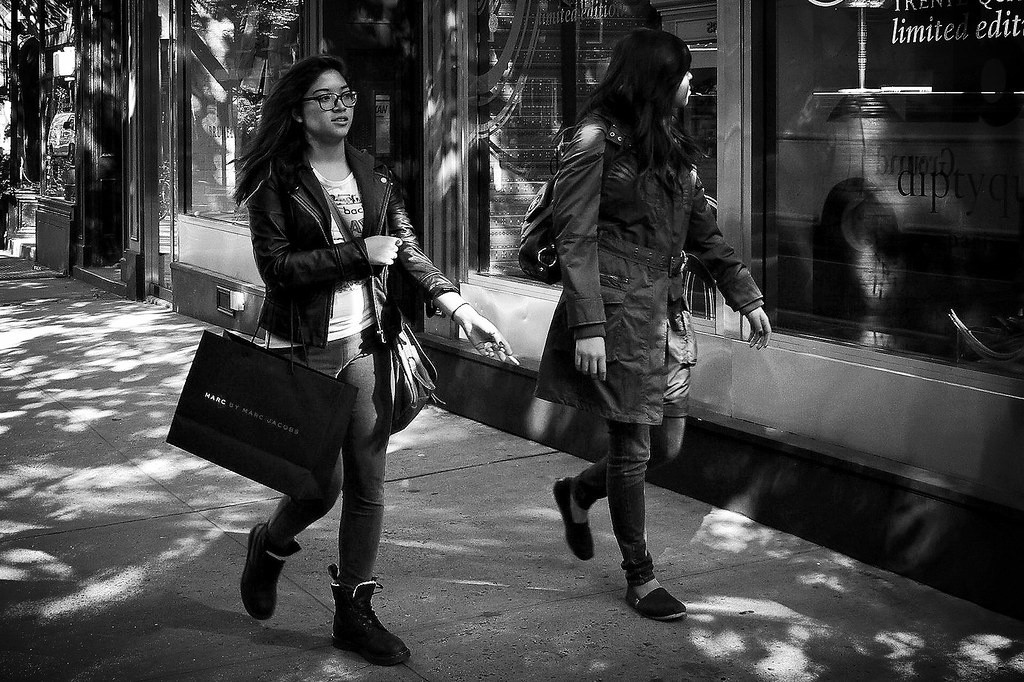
[500, 346, 520, 368]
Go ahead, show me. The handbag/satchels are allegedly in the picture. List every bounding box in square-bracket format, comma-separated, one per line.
[390, 311, 439, 432]
[517, 114, 611, 287]
[165, 293, 360, 503]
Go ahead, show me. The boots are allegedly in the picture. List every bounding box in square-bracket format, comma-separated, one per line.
[240, 516, 302, 621]
[327, 564, 411, 666]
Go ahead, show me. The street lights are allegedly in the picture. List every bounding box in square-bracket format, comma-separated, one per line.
[65, 77, 76, 105]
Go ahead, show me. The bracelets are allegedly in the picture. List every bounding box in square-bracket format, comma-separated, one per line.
[449, 303, 471, 323]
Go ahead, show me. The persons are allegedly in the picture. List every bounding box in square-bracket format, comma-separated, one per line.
[534, 29, 772, 622]
[233, 54, 514, 662]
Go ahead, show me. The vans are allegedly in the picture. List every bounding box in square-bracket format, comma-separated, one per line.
[771, 86, 1024, 254]
[46, 113, 77, 160]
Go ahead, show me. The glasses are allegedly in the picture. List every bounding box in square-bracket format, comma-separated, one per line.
[299, 90, 360, 111]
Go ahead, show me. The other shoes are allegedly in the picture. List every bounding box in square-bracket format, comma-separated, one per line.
[553, 476, 595, 560]
[625, 585, 686, 622]
